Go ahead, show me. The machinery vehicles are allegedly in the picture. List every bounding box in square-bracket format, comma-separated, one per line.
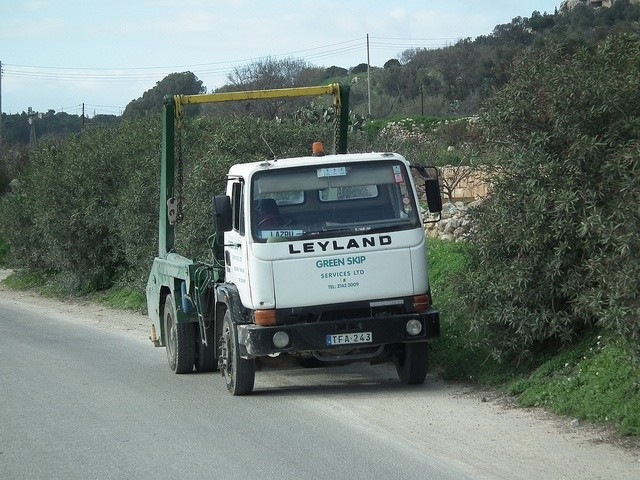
[145, 82, 443, 395]
